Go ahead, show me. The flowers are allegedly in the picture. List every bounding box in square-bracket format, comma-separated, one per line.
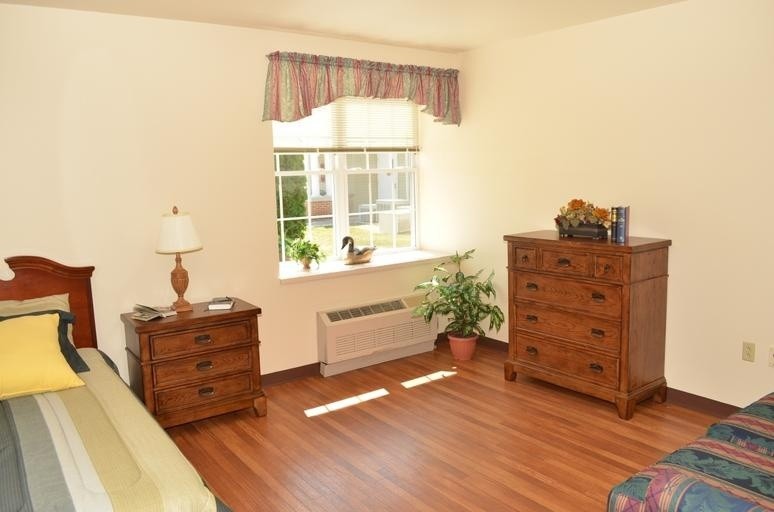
[554, 200, 611, 231]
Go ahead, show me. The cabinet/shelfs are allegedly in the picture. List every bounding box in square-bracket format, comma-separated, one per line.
[504, 232, 672, 420]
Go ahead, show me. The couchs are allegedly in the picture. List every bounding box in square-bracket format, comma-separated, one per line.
[606, 392, 774, 512]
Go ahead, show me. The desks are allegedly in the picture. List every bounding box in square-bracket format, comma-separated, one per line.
[376, 199, 408, 211]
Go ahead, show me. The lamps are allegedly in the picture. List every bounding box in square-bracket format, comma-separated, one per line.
[155, 208, 203, 313]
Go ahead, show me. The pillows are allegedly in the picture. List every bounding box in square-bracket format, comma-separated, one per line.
[0, 293, 91, 400]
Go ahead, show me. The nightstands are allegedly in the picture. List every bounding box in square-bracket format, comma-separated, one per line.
[120, 298, 267, 430]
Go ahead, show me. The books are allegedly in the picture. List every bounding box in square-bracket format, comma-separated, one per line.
[131, 303, 177, 322]
[611, 207, 617, 244]
[208, 296, 235, 311]
[616, 204, 632, 242]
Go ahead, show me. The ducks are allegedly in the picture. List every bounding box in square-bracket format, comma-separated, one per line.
[341, 236, 376, 265]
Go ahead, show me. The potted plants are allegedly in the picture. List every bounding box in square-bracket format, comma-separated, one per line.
[290, 237, 325, 270]
[413, 249, 504, 362]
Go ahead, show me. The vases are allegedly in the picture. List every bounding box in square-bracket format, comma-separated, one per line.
[555, 219, 604, 241]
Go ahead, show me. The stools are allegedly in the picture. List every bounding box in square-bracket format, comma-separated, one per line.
[359, 204, 410, 234]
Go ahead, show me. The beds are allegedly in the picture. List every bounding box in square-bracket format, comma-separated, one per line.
[0, 256, 237, 511]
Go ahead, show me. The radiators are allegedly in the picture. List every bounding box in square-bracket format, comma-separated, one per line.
[316, 293, 438, 378]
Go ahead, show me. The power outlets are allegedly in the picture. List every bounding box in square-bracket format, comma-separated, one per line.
[742, 342, 755, 362]
